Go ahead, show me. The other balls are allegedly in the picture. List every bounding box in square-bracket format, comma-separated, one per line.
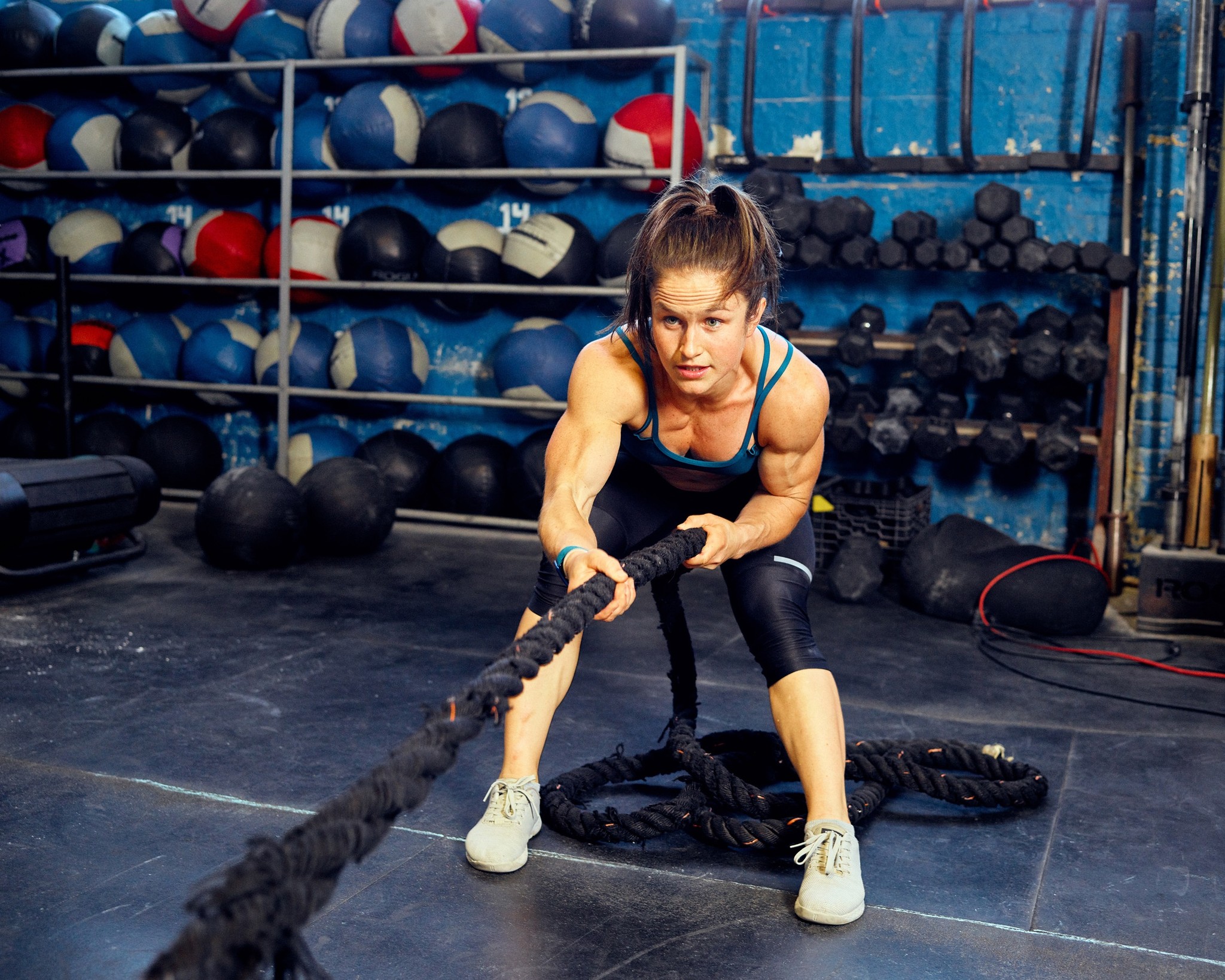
[0, 0, 707, 564]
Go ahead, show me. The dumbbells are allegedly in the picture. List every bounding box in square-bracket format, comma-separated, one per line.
[742, 161, 1136, 479]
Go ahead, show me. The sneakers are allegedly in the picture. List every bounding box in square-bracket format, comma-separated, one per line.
[464, 772, 543, 872]
[790, 820, 865, 926]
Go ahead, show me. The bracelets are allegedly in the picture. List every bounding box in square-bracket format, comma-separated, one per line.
[552, 546, 589, 585]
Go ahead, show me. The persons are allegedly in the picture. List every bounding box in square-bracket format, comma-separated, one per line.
[465, 181, 866, 925]
